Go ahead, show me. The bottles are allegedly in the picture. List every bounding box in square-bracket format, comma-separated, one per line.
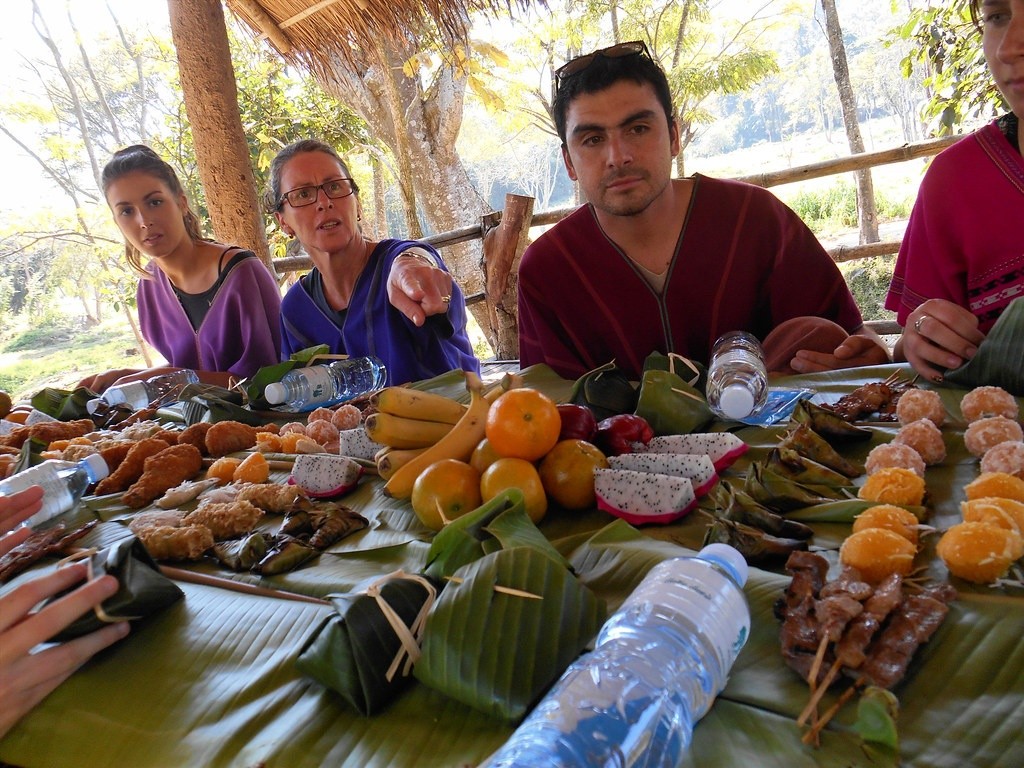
[0, 452, 109, 543]
[87, 369, 200, 415]
[470, 541, 754, 768]
[265, 356, 387, 412]
[705, 330, 769, 422]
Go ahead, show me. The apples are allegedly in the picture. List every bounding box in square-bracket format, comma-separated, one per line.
[555, 403, 598, 443]
[597, 414, 653, 457]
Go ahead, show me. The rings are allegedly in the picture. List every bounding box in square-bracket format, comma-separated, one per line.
[915, 316, 927, 332]
[442, 295, 451, 303]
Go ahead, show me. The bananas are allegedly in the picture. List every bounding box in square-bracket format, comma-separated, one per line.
[365, 370, 523, 499]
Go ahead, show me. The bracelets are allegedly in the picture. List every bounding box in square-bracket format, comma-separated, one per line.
[392, 251, 435, 265]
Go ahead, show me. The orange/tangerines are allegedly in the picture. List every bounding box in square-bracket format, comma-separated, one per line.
[412, 388, 608, 533]
[0, 391, 34, 425]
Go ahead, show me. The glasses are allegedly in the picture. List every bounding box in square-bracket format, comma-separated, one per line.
[554, 40, 656, 98]
[274, 178, 355, 210]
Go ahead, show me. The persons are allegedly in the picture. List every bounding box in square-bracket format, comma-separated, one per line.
[517, 47, 895, 380]
[74, 145, 283, 393]
[885, 0, 1024, 383]
[0, 486, 129, 737]
[268, 141, 484, 380]
